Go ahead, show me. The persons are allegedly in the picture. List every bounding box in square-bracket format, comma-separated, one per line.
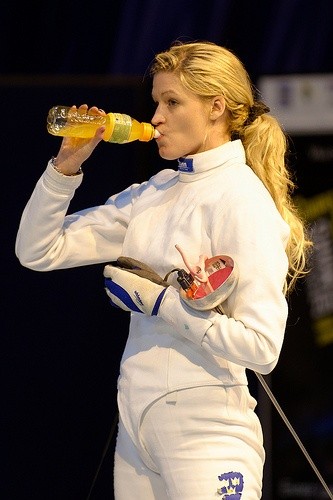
[15, 43, 312, 500]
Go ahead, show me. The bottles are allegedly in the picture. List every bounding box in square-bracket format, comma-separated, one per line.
[47, 106, 160, 144]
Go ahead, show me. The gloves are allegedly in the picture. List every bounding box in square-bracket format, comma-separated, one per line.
[102, 255, 169, 320]
